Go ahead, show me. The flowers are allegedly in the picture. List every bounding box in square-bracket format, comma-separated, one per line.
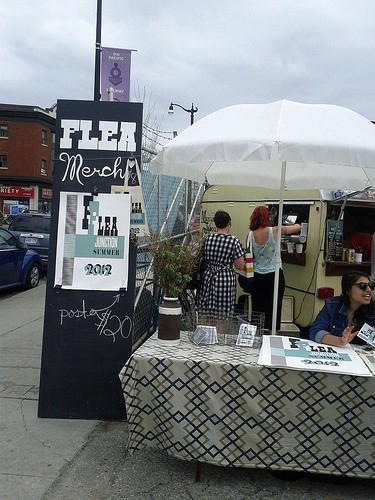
[134, 222, 206, 298]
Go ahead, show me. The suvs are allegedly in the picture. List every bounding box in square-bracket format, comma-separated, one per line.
[7, 210, 51, 277]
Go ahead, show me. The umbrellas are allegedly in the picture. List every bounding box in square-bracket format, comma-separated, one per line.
[150, 99, 375, 337]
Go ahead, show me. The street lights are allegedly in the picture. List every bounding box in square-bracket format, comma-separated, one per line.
[168, 101, 198, 215]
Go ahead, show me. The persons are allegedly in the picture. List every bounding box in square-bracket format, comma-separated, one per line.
[195, 211, 244, 332]
[245, 206, 301, 336]
[42, 210, 51, 215]
[309, 270, 375, 347]
[22, 208, 29, 213]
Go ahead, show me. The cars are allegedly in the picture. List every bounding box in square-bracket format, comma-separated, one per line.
[0, 229, 42, 290]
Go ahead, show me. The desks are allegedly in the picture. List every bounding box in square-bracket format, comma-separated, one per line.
[118, 329, 375, 482]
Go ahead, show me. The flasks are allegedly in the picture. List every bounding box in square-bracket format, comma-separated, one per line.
[157, 293, 181, 347]
[291, 234, 306, 243]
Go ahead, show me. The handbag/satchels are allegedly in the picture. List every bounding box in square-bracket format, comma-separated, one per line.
[233, 231, 254, 279]
[183, 258, 205, 289]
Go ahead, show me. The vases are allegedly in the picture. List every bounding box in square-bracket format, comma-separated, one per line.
[158, 296, 182, 340]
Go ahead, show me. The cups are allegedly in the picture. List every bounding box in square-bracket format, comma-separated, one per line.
[355, 253, 363, 264]
[296, 243, 303, 253]
[287, 243, 294, 253]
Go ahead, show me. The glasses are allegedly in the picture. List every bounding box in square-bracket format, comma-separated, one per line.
[353, 281, 375, 291]
